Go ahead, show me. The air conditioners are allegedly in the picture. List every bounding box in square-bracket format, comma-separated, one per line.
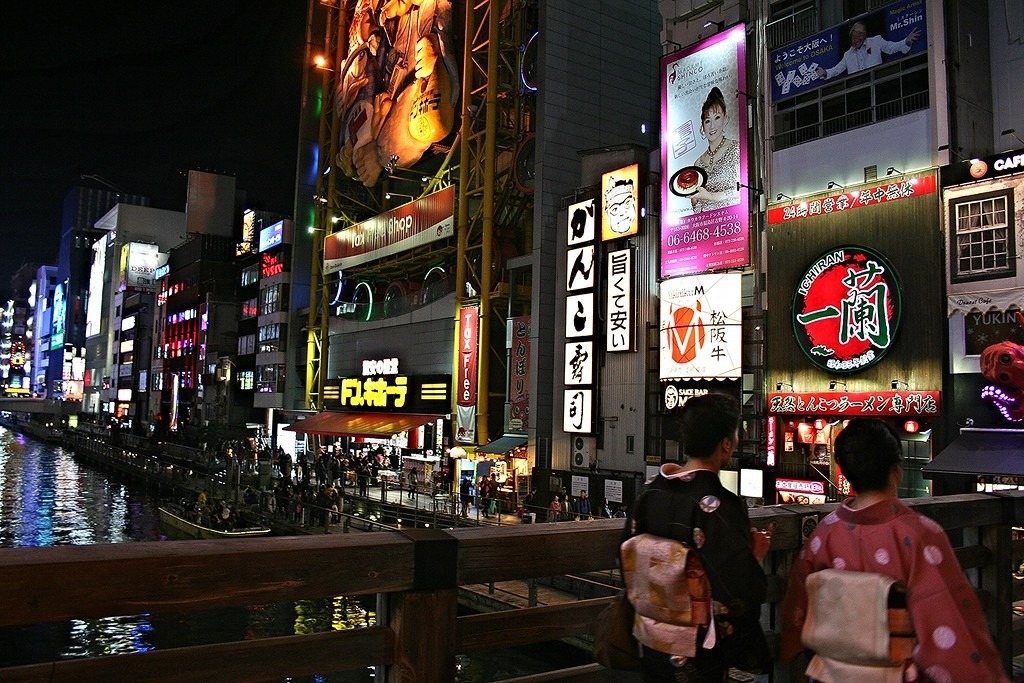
[573, 436, 598, 468]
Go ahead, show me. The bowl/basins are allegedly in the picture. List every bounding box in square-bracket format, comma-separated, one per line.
[669, 166, 707, 197]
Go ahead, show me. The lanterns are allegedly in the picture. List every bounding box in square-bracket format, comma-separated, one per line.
[813, 419, 826, 429]
[905, 421, 919, 432]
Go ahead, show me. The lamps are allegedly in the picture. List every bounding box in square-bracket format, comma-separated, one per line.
[827, 180, 844, 189]
[777, 382, 795, 390]
[308, 227, 329, 236]
[332, 215, 356, 224]
[892, 379, 910, 391]
[386, 193, 414, 204]
[938, 144, 963, 161]
[830, 381, 847, 389]
[317, 194, 329, 203]
[423, 174, 449, 185]
[639, 123, 662, 137]
[640, 208, 658, 221]
[958, 418, 974, 428]
[775, 192, 792, 201]
[887, 166, 901, 176]
[737, 182, 764, 196]
[704, 18, 726, 30]
[1000, 127, 1024, 148]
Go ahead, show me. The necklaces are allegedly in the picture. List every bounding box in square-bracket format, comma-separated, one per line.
[706, 136, 726, 165]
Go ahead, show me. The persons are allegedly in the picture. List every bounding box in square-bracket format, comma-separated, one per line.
[549, 486, 591, 522]
[601, 498, 627, 519]
[198, 438, 396, 530]
[780, 417, 1008, 683]
[480, 473, 501, 518]
[525, 487, 537, 512]
[617, 393, 773, 683]
[817, 21, 922, 80]
[408, 467, 418, 499]
[684, 86, 740, 214]
[460, 479, 469, 517]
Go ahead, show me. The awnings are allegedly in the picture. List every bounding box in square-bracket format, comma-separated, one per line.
[282, 411, 446, 440]
[473, 434, 528, 455]
[921, 431, 1023, 486]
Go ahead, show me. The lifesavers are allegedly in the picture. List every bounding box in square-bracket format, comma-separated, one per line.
[268, 497, 277, 513]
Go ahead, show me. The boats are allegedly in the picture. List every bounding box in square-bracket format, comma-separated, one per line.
[157, 504, 272, 540]
